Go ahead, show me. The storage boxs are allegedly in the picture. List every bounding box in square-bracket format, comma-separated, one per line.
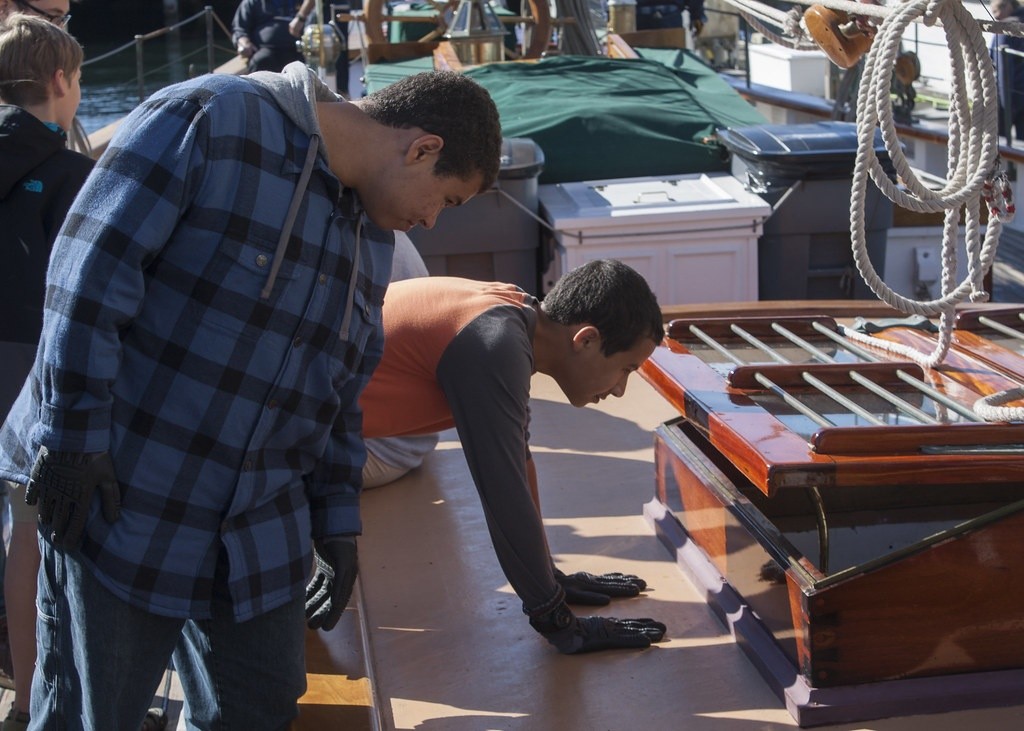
[539, 170, 774, 304]
[749, 39, 829, 125]
[882, 225, 989, 310]
[890, 164, 995, 303]
[723, 118, 908, 301]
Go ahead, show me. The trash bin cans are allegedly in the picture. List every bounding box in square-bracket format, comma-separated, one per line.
[716, 121, 898, 301]
[395, 136, 545, 307]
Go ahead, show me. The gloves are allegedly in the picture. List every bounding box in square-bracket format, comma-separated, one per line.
[553, 566, 647, 606]
[306, 535, 359, 631]
[23, 446, 121, 550]
[532, 602, 668, 655]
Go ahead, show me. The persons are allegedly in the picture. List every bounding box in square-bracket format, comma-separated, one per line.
[613, 0, 706, 41]
[1, 57, 503, 730]
[351, 256, 671, 656]
[1, 14, 103, 728]
[986, 1, 1024, 143]
[231, 0, 308, 76]
[0, 1, 71, 43]
